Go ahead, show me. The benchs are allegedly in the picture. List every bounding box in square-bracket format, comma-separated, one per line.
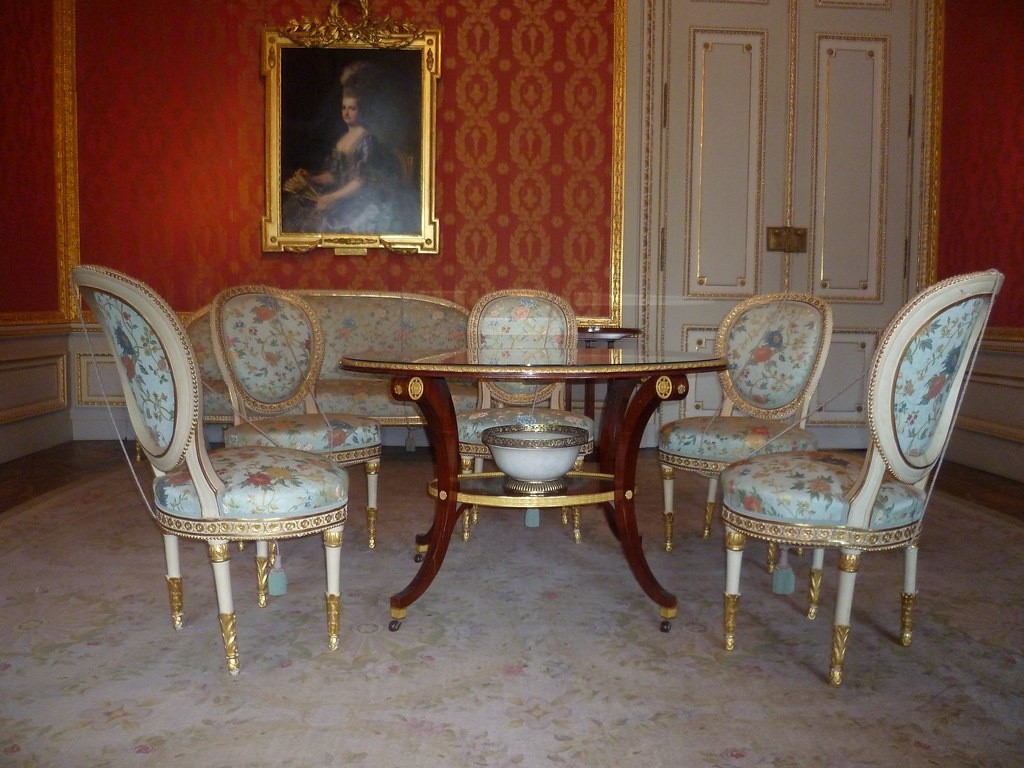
[130, 289, 504, 460]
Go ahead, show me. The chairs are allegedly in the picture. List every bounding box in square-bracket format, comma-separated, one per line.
[73, 258, 350, 674]
[660, 294, 833, 573]
[209, 287, 382, 570]
[454, 290, 593, 544]
[720, 270, 1004, 687]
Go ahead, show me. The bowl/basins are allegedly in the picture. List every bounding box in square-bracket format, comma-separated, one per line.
[480, 422, 589, 496]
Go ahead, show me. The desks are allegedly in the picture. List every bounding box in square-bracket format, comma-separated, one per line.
[565, 326, 643, 461]
[341, 350, 733, 631]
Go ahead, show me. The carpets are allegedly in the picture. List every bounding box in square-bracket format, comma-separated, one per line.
[1, 442, 1023, 768]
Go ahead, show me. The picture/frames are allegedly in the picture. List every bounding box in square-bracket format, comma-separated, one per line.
[260, 0, 440, 254]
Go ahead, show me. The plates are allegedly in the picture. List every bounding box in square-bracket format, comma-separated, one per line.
[571, 323, 644, 349]
